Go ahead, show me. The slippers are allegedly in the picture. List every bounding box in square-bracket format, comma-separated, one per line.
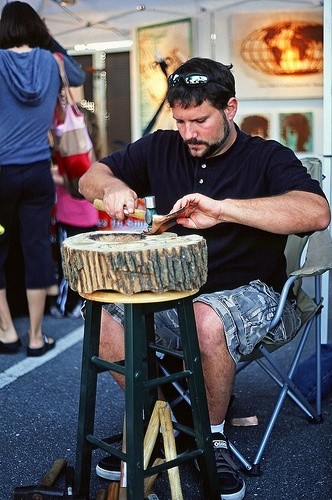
[0, 337, 22, 353]
[27, 335, 55, 356]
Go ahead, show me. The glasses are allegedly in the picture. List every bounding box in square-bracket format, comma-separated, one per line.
[167, 74, 235, 93]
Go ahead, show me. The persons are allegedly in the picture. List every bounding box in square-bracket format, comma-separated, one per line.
[76, 56, 332, 500]
[0, 1, 86, 357]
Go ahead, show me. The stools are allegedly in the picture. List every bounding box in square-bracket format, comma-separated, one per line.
[75, 287, 221, 500]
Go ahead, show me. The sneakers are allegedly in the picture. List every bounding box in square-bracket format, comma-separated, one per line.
[194, 433, 245, 500]
[96, 433, 190, 481]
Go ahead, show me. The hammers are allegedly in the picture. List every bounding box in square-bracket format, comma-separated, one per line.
[14, 458, 66, 500]
[94, 196, 155, 233]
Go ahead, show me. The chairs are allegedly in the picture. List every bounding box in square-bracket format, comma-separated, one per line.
[145, 158, 332, 475]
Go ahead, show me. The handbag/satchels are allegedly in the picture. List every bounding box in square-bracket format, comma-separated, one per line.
[53, 52, 96, 199]
